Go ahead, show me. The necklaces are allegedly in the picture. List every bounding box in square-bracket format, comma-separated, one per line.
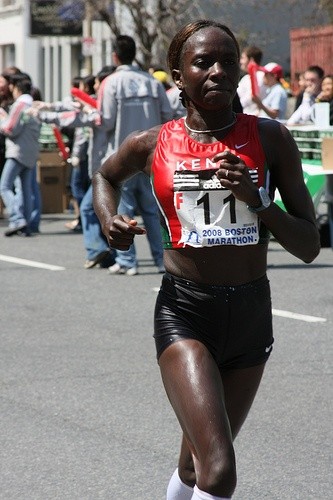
[184, 116, 238, 133]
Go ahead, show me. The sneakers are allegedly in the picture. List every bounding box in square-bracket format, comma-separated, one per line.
[109, 263, 137, 276]
[159, 266, 166, 273]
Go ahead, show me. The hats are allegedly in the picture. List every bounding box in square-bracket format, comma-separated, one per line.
[259, 62, 283, 80]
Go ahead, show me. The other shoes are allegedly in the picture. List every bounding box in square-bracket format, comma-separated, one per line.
[64, 220, 82, 231]
[4, 221, 28, 237]
[19, 226, 38, 234]
[85, 248, 114, 268]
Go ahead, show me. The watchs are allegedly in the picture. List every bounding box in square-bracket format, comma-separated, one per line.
[247, 187, 271, 213]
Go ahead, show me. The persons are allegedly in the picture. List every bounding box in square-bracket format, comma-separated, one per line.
[26, 35, 187, 275]
[237, 45, 333, 130]
[92, 20, 320, 500]
[0, 67, 43, 236]
